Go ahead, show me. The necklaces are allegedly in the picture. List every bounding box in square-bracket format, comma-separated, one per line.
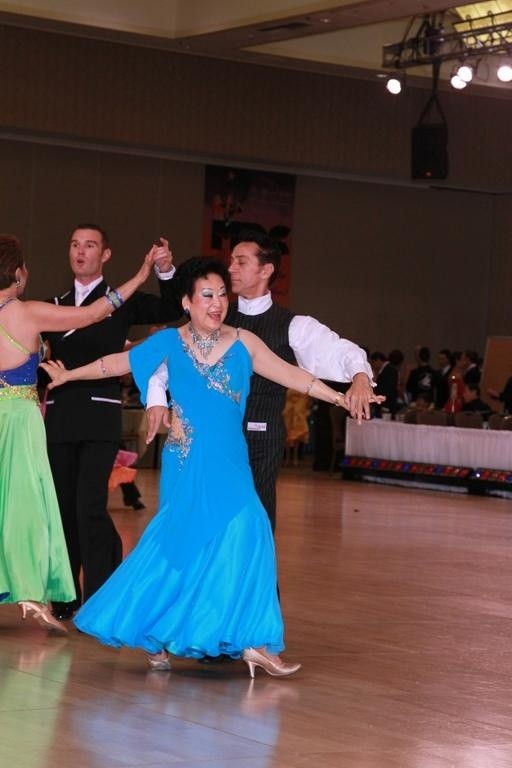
[189, 322, 221, 360]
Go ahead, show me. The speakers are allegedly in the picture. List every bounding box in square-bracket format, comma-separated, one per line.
[409, 120, 448, 182]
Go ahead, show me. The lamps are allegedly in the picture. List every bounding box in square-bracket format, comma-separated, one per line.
[403, 407, 510, 431]
[381, 23, 512, 96]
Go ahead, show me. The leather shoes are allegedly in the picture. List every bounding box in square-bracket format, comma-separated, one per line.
[52, 608, 74, 621]
[124, 499, 145, 510]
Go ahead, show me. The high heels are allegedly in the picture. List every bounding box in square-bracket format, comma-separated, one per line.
[243, 647, 302, 678]
[18, 600, 69, 634]
[146, 653, 171, 671]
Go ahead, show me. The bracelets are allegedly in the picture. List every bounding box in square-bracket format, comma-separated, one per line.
[99, 355, 109, 378]
[105, 285, 125, 309]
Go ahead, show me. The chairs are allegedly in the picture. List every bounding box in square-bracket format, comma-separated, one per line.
[328, 405, 345, 465]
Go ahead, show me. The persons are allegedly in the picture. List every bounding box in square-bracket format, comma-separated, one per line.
[1, 232, 160, 635]
[146, 226, 387, 665]
[26, 225, 181, 622]
[38, 253, 386, 682]
[282, 342, 512, 474]
